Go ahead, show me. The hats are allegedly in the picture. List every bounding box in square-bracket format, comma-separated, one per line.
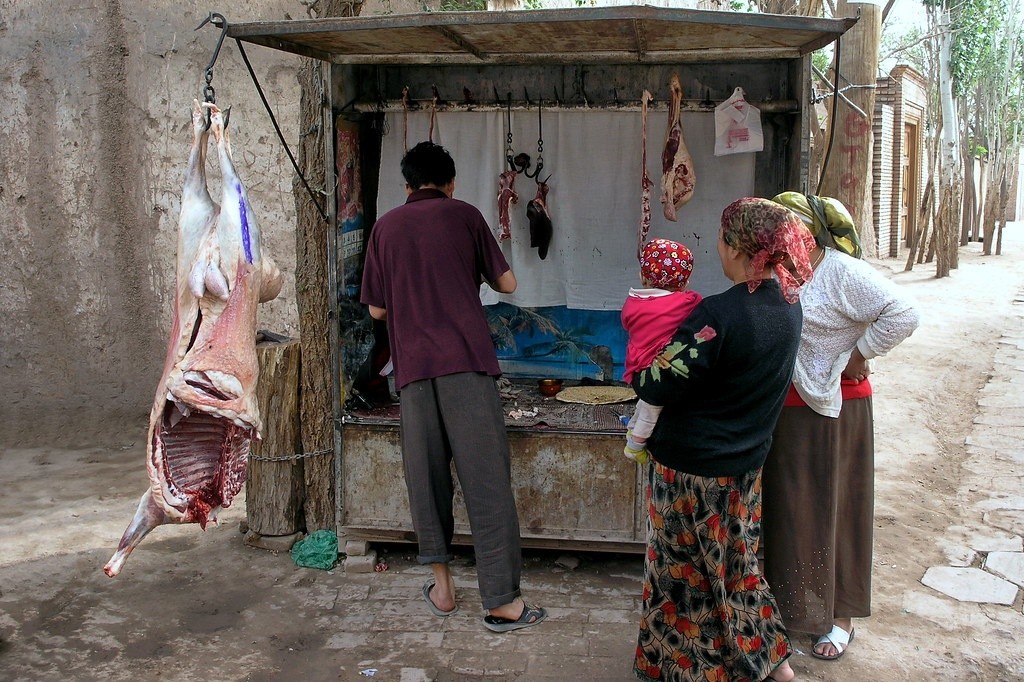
[640, 238, 694, 292]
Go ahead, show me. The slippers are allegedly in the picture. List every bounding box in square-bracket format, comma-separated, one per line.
[812, 624, 855, 660]
[421, 576, 459, 616]
[483, 598, 548, 632]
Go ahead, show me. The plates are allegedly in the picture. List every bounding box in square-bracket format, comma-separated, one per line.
[556, 385, 638, 404]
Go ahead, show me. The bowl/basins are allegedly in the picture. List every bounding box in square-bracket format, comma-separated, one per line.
[537, 378, 563, 397]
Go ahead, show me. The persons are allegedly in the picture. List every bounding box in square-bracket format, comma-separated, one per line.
[360, 141, 549, 632]
[762, 192, 921, 660]
[633, 196, 815, 682]
[620, 238, 702, 465]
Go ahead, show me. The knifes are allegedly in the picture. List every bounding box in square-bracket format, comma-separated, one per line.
[611, 408, 629, 425]
